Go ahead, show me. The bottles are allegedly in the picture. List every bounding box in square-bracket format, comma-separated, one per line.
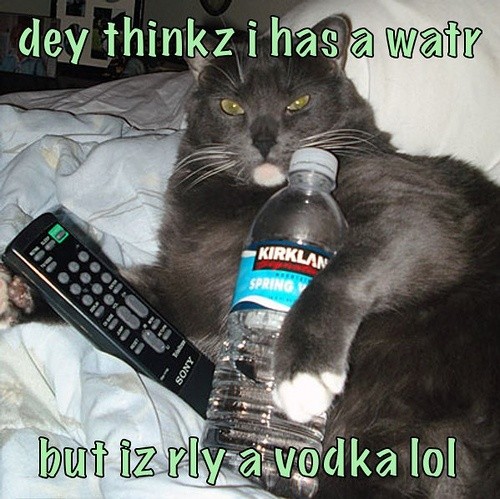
[196, 145, 352, 499]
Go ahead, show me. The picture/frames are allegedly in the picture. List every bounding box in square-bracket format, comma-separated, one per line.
[51, 0, 144, 76]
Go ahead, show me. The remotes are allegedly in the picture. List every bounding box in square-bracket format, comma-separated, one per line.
[1, 211, 240, 433]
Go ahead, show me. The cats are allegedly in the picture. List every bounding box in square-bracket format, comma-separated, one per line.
[0, 13, 500, 498]
